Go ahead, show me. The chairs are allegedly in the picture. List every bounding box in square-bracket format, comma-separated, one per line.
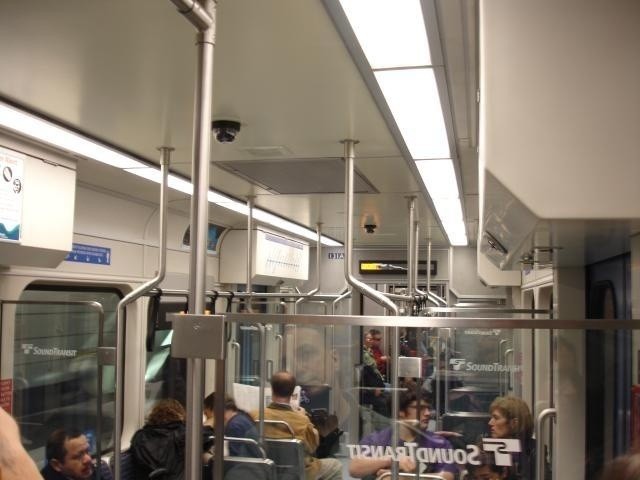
[91, 382, 334, 480]
[376, 370, 534, 480]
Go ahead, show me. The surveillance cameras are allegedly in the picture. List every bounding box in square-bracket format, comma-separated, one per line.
[364, 225, 376, 233]
[211, 120, 240, 143]
